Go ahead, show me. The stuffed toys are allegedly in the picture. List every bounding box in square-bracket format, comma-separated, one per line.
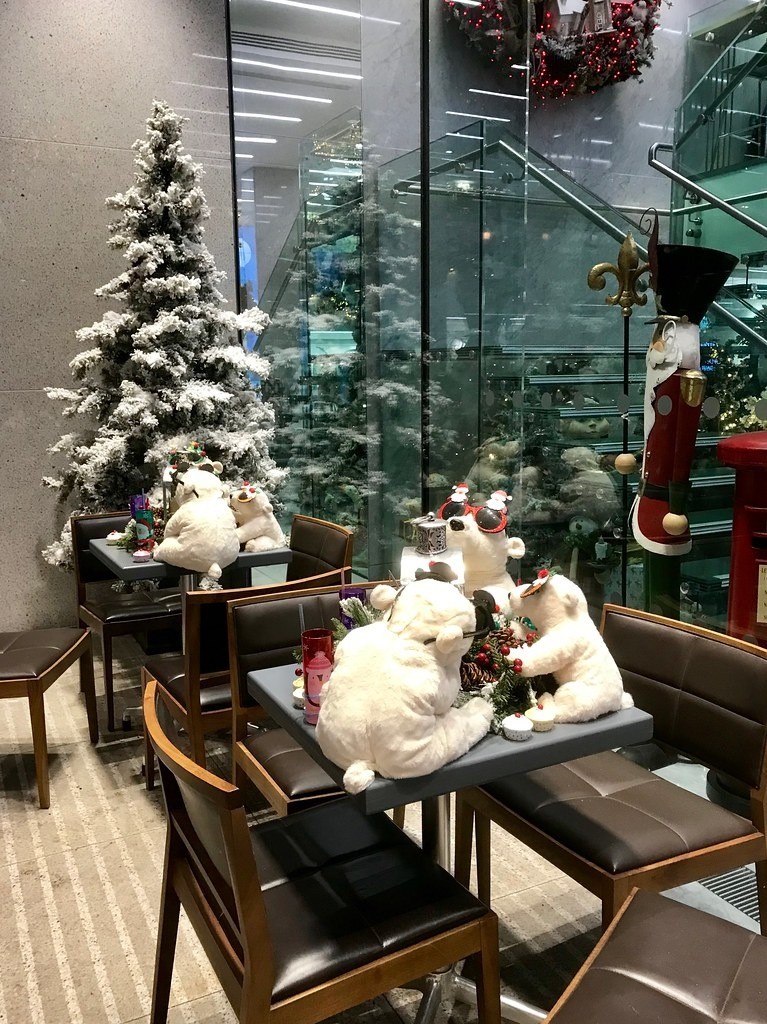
[433, 483, 526, 614]
[315, 578, 493, 792]
[507, 574, 633, 725]
[229, 485, 287, 553]
[152, 469, 240, 578]
[189, 458, 222, 475]
[458, 437, 619, 534]
[106, 488, 171, 545]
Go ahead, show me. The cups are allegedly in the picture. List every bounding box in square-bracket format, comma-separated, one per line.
[293, 677, 306, 707]
[301, 628, 337, 725]
[136, 510, 155, 551]
[130, 494, 147, 520]
[339, 587, 366, 629]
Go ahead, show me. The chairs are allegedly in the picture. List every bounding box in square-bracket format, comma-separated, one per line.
[226, 580, 408, 830]
[0, 627, 100, 810]
[137, 679, 502, 1024]
[454, 600, 767, 935]
[286, 513, 353, 584]
[139, 564, 352, 792]
[68, 512, 181, 748]
[538, 885, 767, 1024]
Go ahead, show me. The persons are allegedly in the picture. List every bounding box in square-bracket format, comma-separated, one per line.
[615, 244, 740, 617]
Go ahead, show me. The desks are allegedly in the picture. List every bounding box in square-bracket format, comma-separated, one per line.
[89, 536, 294, 732]
[246, 663, 656, 1024]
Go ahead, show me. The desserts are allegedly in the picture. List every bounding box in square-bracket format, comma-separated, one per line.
[106, 529, 122, 546]
[133, 548, 150, 563]
[292, 677, 305, 709]
[502, 705, 556, 741]
